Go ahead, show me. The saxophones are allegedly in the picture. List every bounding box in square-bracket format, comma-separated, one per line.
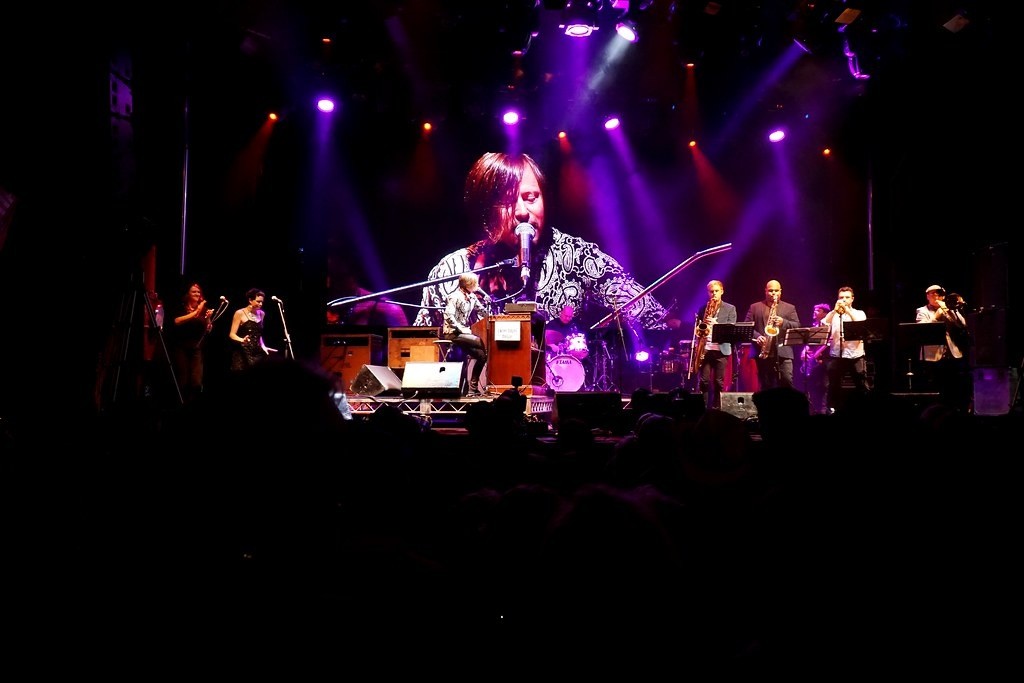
[694, 297, 719, 375]
[760, 295, 780, 358]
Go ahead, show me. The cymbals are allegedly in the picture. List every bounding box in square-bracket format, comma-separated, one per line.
[545, 330, 564, 345]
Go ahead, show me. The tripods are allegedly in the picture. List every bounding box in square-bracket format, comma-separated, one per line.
[589, 341, 620, 391]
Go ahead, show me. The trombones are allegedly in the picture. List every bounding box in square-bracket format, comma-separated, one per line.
[937, 293, 972, 335]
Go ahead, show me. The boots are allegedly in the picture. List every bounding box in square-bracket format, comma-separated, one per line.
[466, 381, 481, 396]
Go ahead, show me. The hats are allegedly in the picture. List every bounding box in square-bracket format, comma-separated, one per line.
[925, 285, 945, 293]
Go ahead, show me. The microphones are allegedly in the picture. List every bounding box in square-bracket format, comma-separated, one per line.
[271, 296, 282, 302]
[476, 287, 490, 298]
[515, 222, 536, 283]
[555, 377, 559, 382]
[220, 296, 229, 304]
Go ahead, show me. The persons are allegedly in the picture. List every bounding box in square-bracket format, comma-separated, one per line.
[443, 272, 490, 396]
[916, 284, 966, 392]
[823, 287, 866, 415]
[172, 282, 213, 385]
[230, 289, 278, 369]
[546, 306, 573, 352]
[330, 246, 409, 326]
[413, 151, 668, 330]
[745, 280, 800, 388]
[800, 303, 830, 411]
[0, 358, 1024, 683]
[698, 280, 737, 410]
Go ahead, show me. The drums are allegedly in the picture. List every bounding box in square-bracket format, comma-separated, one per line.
[564, 332, 589, 359]
[545, 354, 585, 393]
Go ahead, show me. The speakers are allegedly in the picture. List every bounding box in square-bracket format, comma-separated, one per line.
[719, 392, 759, 422]
[319, 327, 469, 397]
[552, 392, 622, 430]
[892, 393, 940, 416]
[968, 308, 1011, 368]
